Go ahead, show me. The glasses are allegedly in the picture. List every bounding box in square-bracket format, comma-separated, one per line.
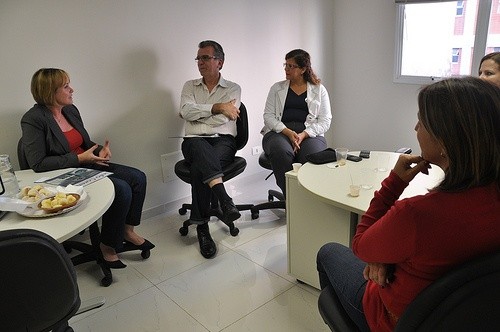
[283, 63, 303, 70]
[194, 55, 219, 62]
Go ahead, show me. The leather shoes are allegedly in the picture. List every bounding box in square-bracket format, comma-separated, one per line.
[197, 225, 216, 258]
[219, 197, 242, 223]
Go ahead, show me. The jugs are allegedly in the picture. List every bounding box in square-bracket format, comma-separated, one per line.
[0, 154, 19, 198]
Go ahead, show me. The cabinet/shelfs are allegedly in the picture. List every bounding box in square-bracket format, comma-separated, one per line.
[285, 168, 351, 292]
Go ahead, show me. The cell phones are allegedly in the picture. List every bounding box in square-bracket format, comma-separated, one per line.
[359, 150, 370, 158]
[347, 155, 362, 162]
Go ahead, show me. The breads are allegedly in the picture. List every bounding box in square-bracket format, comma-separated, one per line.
[22, 185, 76, 208]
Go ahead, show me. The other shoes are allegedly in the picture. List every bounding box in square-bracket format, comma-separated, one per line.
[121, 237, 156, 251]
[93, 241, 128, 270]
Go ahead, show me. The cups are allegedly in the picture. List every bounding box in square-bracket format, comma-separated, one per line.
[292, 163, 302, 171]
[335, 146, 348, 166]
[349, 155, 388, 196]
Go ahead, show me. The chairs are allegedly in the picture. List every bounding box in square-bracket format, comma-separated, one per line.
[174, 102, 255, 236]
[0, 229, 82, 332]
[251, 150, 286, 220]
[317, 250, 500, 332]
[17, 137, 150, 287]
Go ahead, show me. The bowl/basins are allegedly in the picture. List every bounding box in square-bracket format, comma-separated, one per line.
[38, 193, 80, 213]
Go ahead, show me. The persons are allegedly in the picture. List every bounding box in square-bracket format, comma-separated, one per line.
[478, 52, 500, 90]
[260, 49, 332, 200]
[21, 68, 155, 268]
[180, 40, 241, 259]
[318, 75, 500, 332]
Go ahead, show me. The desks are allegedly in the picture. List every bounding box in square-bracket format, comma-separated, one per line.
[0, 167, 115, 315]
[297, 151, 445, 250]
